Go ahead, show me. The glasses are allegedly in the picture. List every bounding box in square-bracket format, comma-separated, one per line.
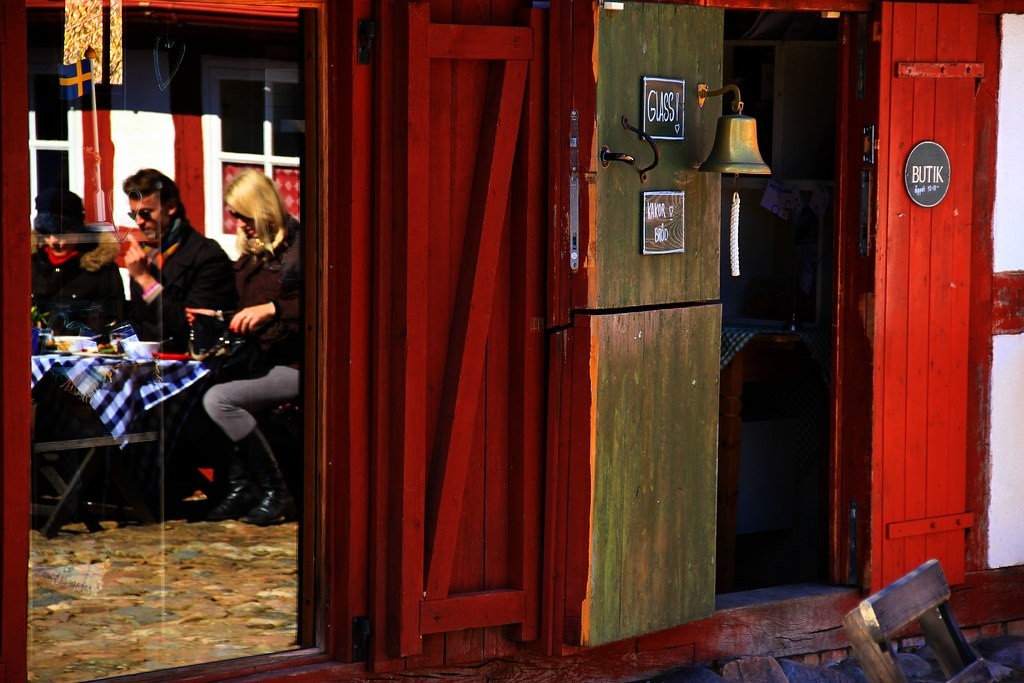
[38, 228, 67, 238]
[229, 211, 252, 222]
[127, 210, 151, 220]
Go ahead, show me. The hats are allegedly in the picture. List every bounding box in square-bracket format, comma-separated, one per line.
[36, 190, 83, 232]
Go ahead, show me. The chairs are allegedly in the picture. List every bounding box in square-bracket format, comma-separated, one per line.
[840, 559, 1024, 683]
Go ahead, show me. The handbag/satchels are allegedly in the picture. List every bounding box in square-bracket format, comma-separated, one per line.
[187, 311, 265, 378]
[53, 309, 105, 336]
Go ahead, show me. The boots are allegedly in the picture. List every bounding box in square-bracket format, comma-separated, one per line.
[235, 425, 297, 524]
[206, 439, 266, 520]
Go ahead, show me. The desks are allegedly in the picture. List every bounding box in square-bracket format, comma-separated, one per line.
[29, 346, 207, 537]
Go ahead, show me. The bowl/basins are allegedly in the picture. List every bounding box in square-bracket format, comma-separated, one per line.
[124, 341, 161, 360]
[54, 336, 93, 352]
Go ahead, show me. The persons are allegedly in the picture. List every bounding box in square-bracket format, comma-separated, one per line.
[30, 168, 302, 524]
